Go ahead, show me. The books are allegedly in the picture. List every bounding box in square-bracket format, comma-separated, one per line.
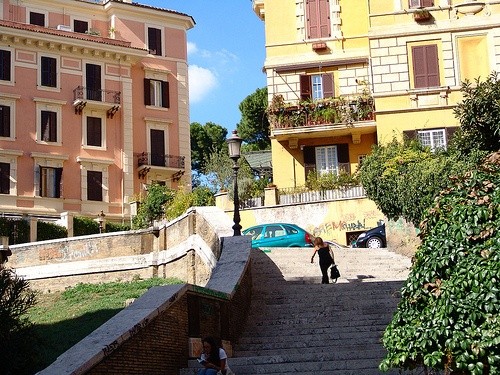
[197, 359, 208, 365]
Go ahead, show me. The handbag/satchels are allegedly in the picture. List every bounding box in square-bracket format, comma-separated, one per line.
[330, 264, 340, 283]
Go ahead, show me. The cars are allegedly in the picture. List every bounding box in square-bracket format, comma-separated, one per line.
[356, 223, 386, 248]
[237, 221, 315, 248]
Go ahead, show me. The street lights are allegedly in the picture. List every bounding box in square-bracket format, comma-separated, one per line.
[97, 210, 106, 234]
[225, 127, 244, 235]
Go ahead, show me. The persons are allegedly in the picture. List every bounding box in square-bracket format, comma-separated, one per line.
[196, 334, 227, 375]
[311, 237, 335, 284]
